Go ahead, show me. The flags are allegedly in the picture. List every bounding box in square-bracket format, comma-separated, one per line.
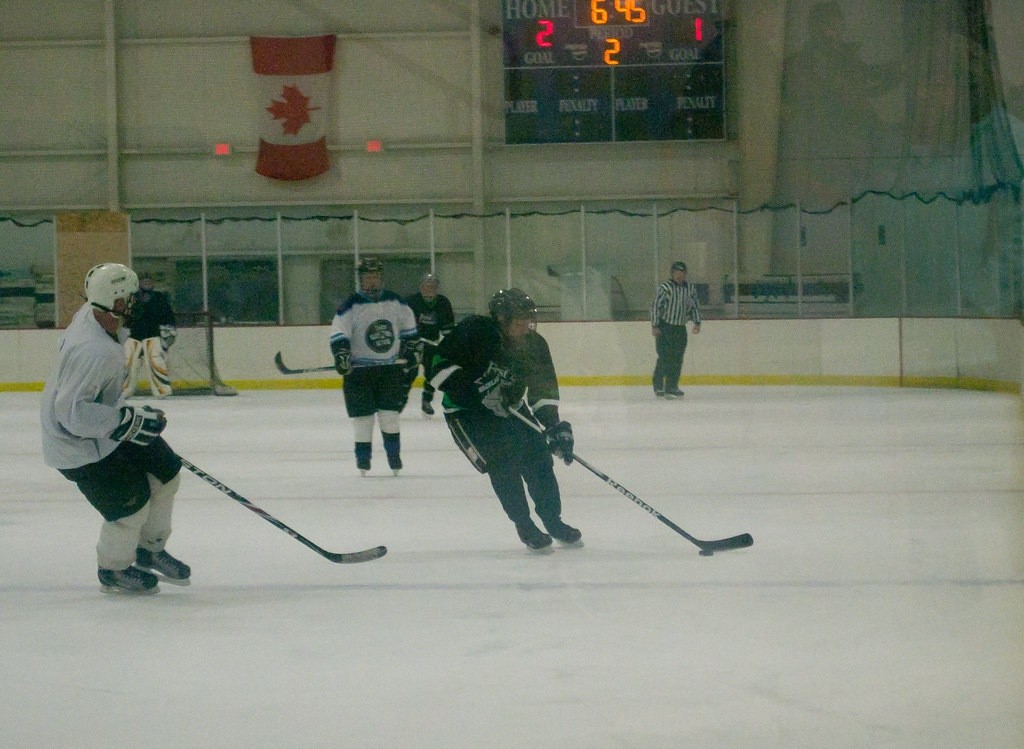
[248, 33, 331, 182]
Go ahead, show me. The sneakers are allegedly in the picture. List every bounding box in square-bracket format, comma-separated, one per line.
[131, 546, 192, 586]
[535, 497, 585, 549]
[515, 517, 554, 553]
[356, 458, 371, 477]
[97, 564, 160, 596]
[420, 400, 434, 420]
[664, 387, 684, 398]
[387, 455, 402, 476]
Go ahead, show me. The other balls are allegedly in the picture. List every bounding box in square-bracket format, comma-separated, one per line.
[699, 550, 713, 556]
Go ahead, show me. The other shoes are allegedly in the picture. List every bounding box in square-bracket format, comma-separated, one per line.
[653, 386, 665, 398]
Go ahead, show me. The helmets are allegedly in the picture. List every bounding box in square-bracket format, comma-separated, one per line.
[358, 256, 384, 292]
[671, 261, 688, 286]
[419, 272, 440, 302]
[84, 262, 140, 318]
[139, 272, 155, 294]
[489, 287, 538, 352]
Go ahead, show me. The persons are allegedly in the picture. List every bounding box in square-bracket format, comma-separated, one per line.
[331, 256, 421, 478]
[39, 262, 193, 596]
[433, 286, 584, 554]
[397, 268, 455, 419]
[650, 263, 702, 401]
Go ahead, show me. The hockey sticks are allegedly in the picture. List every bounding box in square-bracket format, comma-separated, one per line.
[508, 406, 755, 552]
[274, 351, 408, 376]
[177, 451, 388, 564]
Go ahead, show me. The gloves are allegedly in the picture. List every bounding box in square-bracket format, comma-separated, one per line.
[472, 366, 524, 418]
[542, 420, 574, 467]
[331, 339, 353, 376]
[399, 336, 424, 387]
[110, 405, 167, 446]
